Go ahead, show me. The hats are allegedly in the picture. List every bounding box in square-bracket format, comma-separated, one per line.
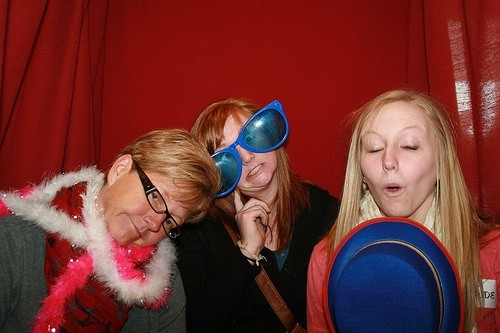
[322, 217, 464, 333]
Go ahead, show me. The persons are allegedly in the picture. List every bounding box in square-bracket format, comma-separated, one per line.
[304, 89, 500, 333]
[164, 96, 340, 333]
[1, 125, 222, 333]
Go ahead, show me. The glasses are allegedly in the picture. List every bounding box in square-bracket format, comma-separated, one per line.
[209, 100, 288, 199]
[132, 160, 181, 239]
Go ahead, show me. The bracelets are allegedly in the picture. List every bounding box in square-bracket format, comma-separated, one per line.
[233, 238, 267, 264]
